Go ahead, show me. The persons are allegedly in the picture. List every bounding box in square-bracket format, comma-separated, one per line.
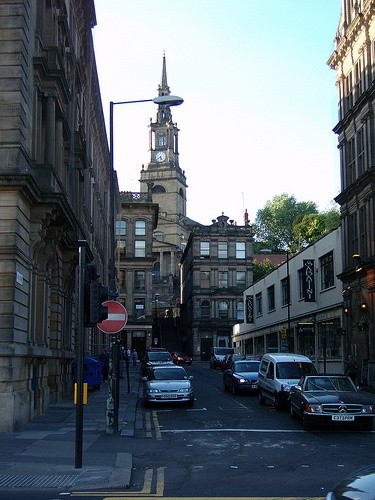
[132, 349, 137, 368]
[344, 355, 357, 384]
[126, 348, 131, 368]
[100, 348, 110, 383]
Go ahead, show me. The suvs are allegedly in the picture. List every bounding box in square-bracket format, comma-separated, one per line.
[140, 347, 166, 361]
[140, 351, 175, 377]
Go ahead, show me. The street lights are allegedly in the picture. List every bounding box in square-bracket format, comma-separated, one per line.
[106, 95, 185, 433]
[154, 293, 160, 347]
[258, 249, 291, 351]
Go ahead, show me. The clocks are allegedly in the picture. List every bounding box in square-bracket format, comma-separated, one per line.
[155, 151, 166, 163]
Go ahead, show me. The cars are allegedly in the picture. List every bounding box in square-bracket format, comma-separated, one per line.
[138, 365, 194, 408]
[221, 353, 254, 371]
[223, 360, 261, 396]
[287, 374, 375, 432]
[324, 464, 375, 500]
[169, 351, 193, 365]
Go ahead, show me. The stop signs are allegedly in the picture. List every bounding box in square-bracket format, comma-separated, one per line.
[97, 301, 127, 334]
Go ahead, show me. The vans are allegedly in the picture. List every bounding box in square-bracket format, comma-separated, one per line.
[257, 352, 319, 413]
[210, 347, 234, 370]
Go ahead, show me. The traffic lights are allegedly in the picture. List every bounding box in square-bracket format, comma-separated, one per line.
[86, 281, 120, 327]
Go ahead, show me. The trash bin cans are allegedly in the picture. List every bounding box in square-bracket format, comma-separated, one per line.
[70, 356, 104, 391]
